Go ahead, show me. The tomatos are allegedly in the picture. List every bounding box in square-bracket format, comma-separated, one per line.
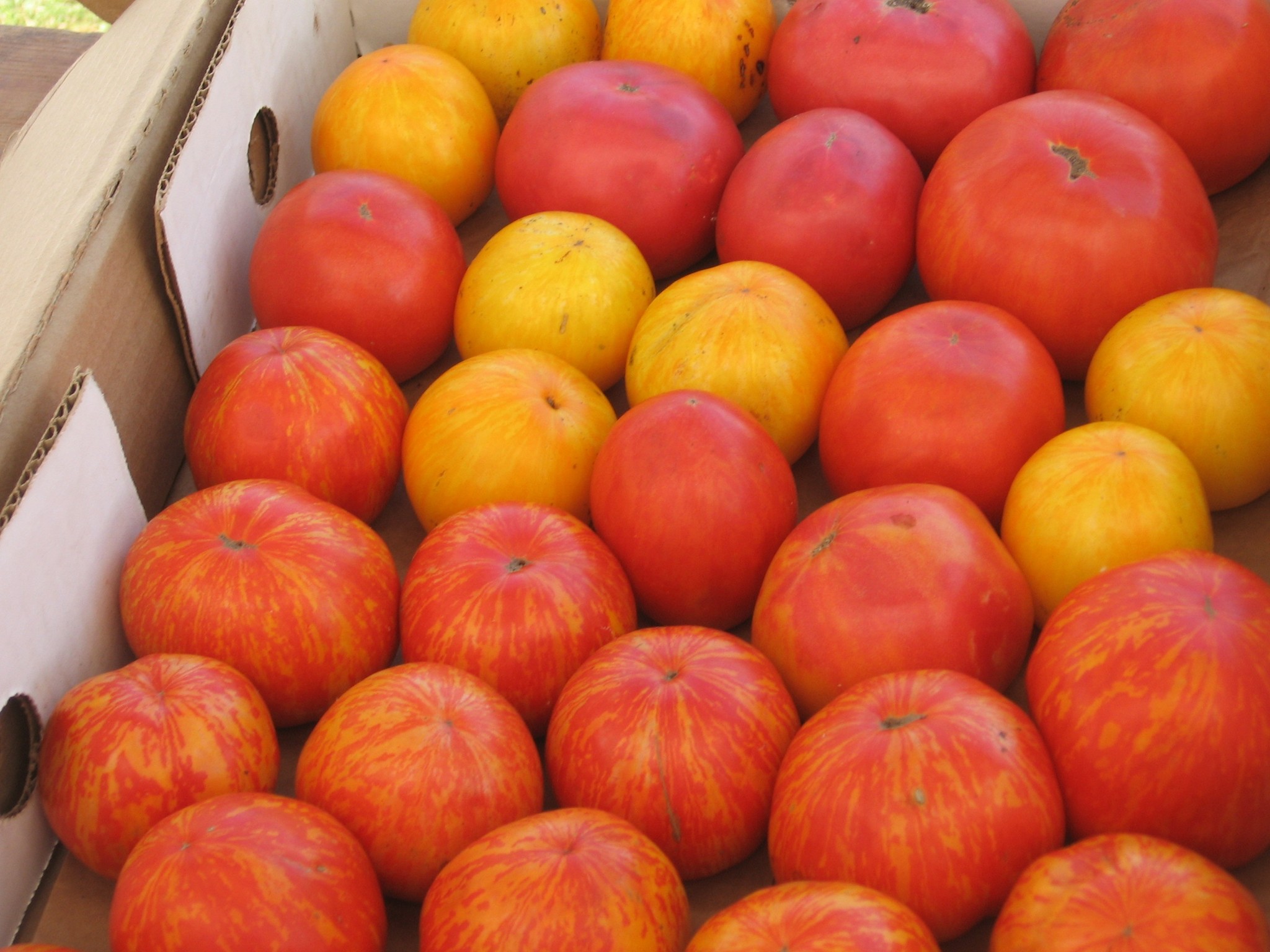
[41, 1, 1270, 950]
